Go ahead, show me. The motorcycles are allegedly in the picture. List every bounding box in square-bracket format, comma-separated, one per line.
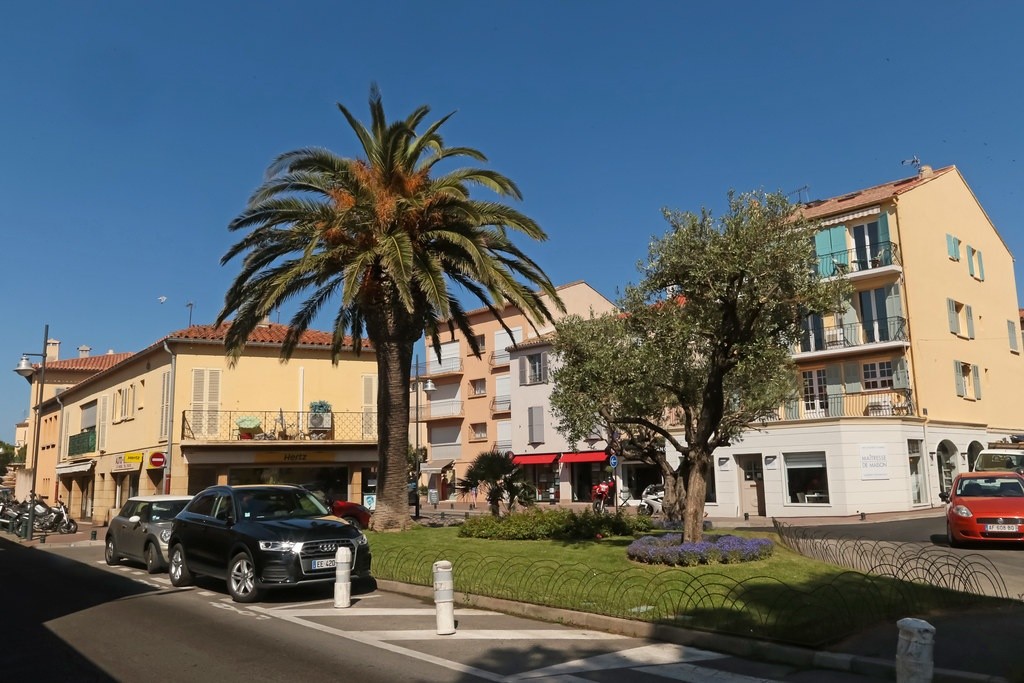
[636, 484, 665, 518]
[0, 487, 78, 538]
[590, 477, 615, 517]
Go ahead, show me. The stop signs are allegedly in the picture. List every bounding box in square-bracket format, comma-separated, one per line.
[151, 452, 165, 467]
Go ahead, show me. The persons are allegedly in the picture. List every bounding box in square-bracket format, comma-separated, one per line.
[140, 505, 153, 521]
[218, 496, 257, 521]
[364, 496, 375, 510]
[469, 487, 478, 509]
[442, 475, 448, 499]
[603, 476, 615, 506]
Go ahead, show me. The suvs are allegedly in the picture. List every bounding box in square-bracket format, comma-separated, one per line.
[166, 483, 373, 604]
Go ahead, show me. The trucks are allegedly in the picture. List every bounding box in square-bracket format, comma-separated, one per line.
[961, 443, 1024, 489]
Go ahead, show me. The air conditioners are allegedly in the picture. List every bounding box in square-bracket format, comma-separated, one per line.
[308, 412, 331, 428]
[868, 396, 890, 415]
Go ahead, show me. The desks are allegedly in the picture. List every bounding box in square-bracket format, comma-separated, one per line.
[804, 495, 829, 503]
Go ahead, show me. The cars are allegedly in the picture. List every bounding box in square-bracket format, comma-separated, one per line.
[937, 470, 1024, 547]
[103, 494, 196, 574]
[324, 498, 372, 530]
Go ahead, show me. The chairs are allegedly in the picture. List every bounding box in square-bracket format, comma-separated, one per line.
[232, 415, 261, 437]
[830, 249, 890, 274]
[965, 483, 1020, 495]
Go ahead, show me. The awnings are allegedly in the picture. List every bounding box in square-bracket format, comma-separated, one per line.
[558, 452, 608, 462]
[512, 454, 558, 464]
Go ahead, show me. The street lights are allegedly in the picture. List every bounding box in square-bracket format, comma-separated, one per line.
[11, 323, 51, 540]
[410, 355, 437, 520]
[186, 301, 194, 328]
